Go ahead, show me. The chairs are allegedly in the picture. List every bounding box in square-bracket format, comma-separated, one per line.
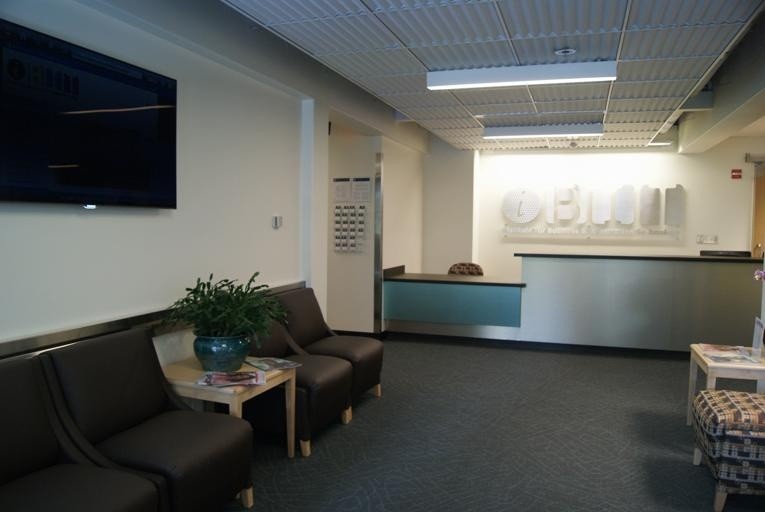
[0, 337, 169, 512]
[447, 263, 483, 275]
[274, 288, 383, 425]
[36, 329, 253, 512]
[250, 291, 354, 457]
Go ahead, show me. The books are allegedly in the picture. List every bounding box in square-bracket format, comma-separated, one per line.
[245, 357, 303, 371]
[196, 371, 266, 387]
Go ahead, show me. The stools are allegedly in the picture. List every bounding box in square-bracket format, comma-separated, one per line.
[690, 389, 765, 512]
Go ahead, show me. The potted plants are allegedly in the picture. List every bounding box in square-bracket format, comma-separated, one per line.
[159, 272, 287, 374]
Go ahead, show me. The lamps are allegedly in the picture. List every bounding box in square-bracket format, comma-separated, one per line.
[481, 122, 603, 140]
[426, 60, 617, 91]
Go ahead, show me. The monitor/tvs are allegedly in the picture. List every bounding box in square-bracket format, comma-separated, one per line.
[0, 18, 177, 210]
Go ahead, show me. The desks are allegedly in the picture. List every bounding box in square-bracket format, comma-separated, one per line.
[685, 343, 765, 466]
[163, 352, 297, 508]
